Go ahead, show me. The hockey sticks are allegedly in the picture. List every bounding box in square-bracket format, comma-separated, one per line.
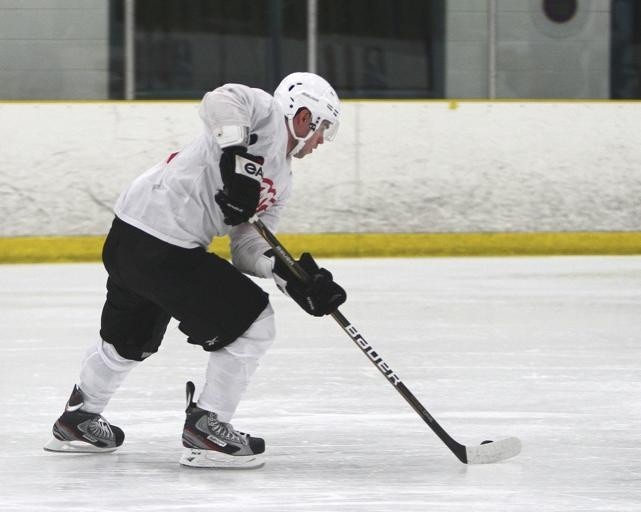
[248, 214, 522, 464]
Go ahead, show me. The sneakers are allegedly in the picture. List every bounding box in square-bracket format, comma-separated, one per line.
[181, 381, 265, 457]
[52, 383, 125, 449]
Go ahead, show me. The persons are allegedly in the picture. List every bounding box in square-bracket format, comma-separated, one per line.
[43, 69, 347, 471]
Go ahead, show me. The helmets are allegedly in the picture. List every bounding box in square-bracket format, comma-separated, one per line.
[273, 71, 343, 142]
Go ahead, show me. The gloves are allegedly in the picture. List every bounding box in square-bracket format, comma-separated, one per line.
[260, 248, 347, 317]
[214, 150, 264, 227]
